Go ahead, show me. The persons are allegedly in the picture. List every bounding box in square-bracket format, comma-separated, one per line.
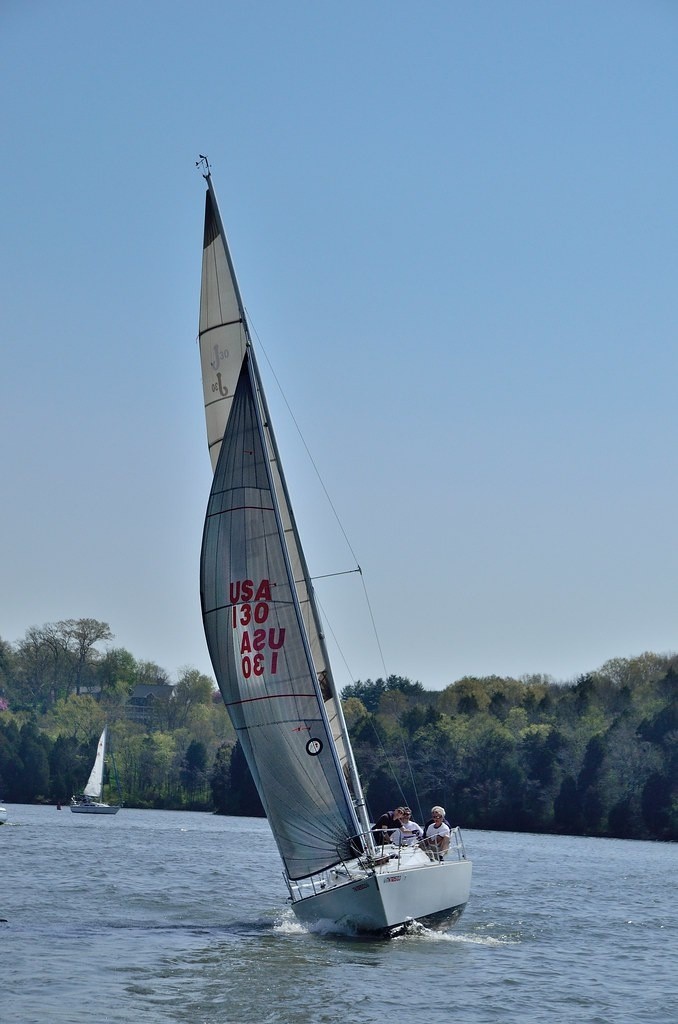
[422, 806, 451, 839]
[70, 795, 76, 805]
[371, 807, 420, 846]
[390, 807, 423, 846]
[82, 794, 89, 806]
[420, 809, 449, 860]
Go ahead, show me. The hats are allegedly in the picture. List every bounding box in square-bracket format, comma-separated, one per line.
[398, 806, 405, 813]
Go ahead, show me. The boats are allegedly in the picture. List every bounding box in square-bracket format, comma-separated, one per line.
[0, 806, 11, 825]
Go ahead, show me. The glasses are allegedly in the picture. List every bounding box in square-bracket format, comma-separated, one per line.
[433, 815, 442, 820]
[404, 813, 410, 816]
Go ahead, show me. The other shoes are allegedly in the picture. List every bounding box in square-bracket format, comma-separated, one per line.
[440, 855, 444, 861]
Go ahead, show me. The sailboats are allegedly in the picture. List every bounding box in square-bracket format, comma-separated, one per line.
[63, 722, 126, 813]
[187, 150, 480, 942]
